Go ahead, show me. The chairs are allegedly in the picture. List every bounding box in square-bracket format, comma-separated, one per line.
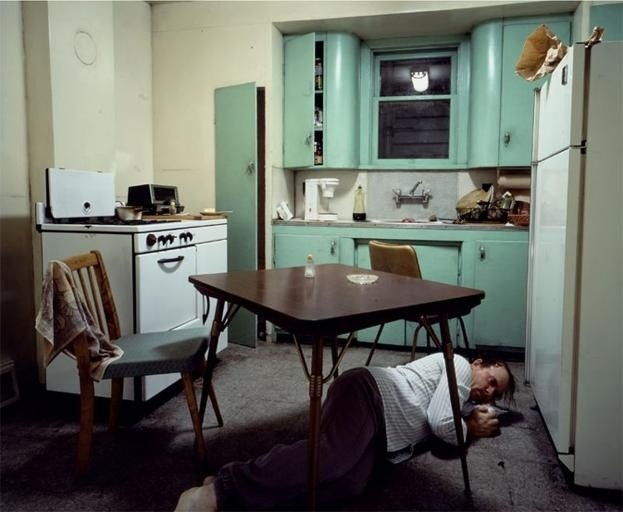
[30, 245, 224, 491]
[363, 237, 474, 371]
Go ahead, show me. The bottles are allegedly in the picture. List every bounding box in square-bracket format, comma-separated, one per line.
[351, 183, 366, 221]
[302, 252, 316, 279]
[314, 143, 322, 165]
[169, 197, 176, 217]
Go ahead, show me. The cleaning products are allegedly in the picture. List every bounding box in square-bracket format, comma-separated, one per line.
[353, 186, 366, 221]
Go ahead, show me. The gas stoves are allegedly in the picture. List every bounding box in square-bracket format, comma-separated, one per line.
[53, 217, 194, 253]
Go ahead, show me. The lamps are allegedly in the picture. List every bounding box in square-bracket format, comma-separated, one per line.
[408, 65, 429, 92]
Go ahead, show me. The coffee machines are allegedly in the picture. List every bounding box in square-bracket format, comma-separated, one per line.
[300, 178, 339, 221]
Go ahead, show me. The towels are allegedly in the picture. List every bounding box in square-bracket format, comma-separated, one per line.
[32, 258, 125, 388]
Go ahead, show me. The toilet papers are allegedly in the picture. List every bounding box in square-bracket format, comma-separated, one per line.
[498, 176, 531, 203]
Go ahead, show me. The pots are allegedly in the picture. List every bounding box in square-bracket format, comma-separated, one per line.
[114, 204, 151, 222]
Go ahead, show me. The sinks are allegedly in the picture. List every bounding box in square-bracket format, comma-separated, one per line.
[373, 218, 443, 225]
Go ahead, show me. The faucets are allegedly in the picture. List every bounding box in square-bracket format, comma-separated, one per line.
[409, 181, 422, 195]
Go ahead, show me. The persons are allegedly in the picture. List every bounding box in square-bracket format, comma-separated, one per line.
[171, 346, 518, 512]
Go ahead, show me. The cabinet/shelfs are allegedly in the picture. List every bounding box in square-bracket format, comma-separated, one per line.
[41, 245, 196, 402]
[273, 233, 341, 275]
[468, 235, 530, 348]
[468, 17, 571, 170]
[195, 239, 228, 358]
[281, 30, 363, 169]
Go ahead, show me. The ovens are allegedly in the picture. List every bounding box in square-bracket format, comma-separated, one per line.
[133, 244, 197, 401]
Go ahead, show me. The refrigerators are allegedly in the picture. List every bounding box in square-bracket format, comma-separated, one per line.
[522, 34, 623, 496]
